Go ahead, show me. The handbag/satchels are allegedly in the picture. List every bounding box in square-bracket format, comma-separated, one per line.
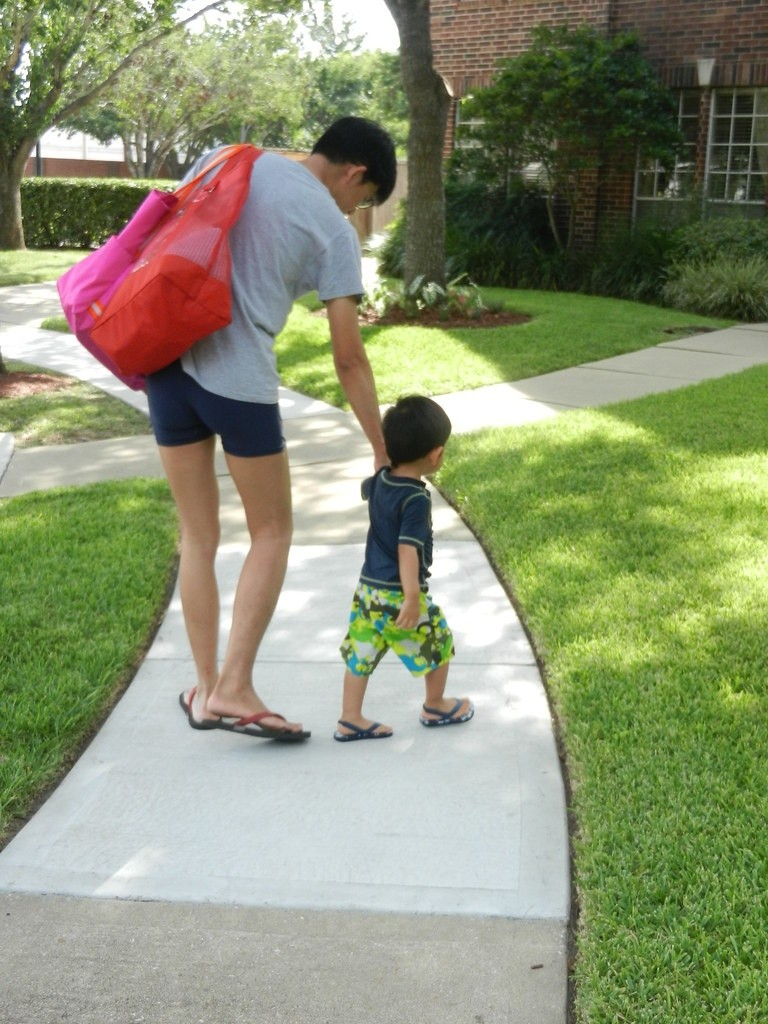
[57, 144, 263, 393]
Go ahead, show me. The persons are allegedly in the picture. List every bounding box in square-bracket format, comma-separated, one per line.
[141, 116, 400, 742]
[330, 396, 477, 743]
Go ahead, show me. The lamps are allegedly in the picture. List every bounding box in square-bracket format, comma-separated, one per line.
[696, 58, 713, 85]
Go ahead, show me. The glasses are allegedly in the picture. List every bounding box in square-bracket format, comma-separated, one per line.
[357, 197, 374, 210]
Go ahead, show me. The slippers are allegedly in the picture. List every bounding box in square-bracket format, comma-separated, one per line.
[201, 712, 311, 740]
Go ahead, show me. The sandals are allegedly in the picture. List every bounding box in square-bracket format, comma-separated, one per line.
[180, 686, 223, 729]
[334, 718, 394, 742]
[420, 697, 474, 727]
